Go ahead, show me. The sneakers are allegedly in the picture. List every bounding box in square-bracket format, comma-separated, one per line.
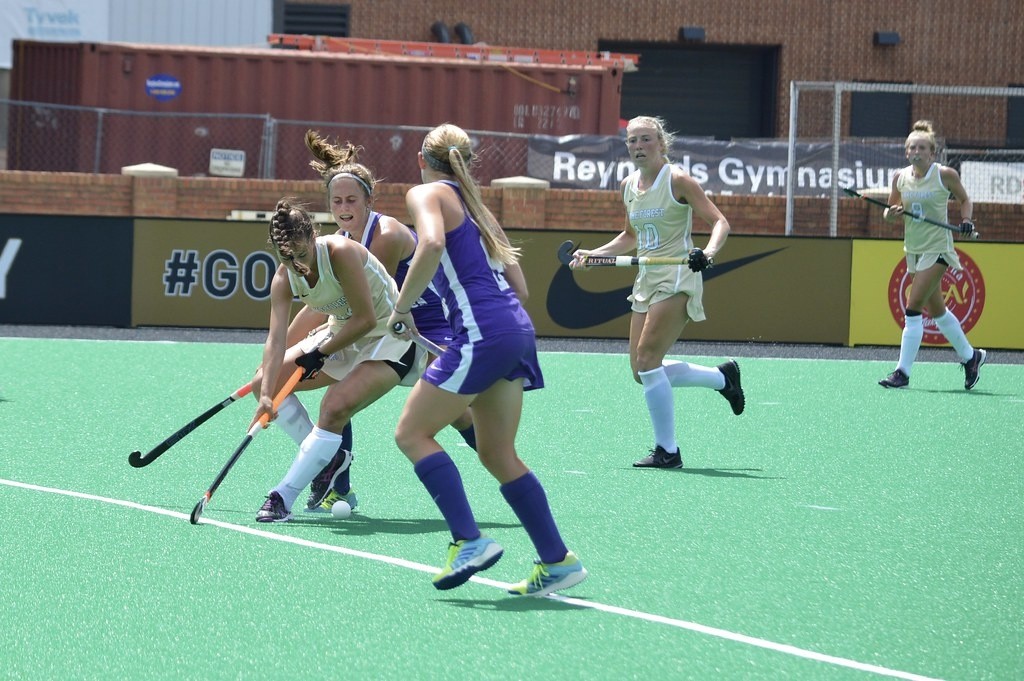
[433, 533, 504, 590]
[632, 445, 683, 468]
[878, 369, 910, 389]
[714, 359, 746, 416]
[307, 447, 354, 509]
[303, 487, 358, 512]
[957, 349, 987, 390]
[508, 550, 588, 598]
[256, 491, 293, 522]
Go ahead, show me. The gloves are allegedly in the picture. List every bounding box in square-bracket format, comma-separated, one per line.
[295, 347, 330, 382]
[688, 247, 708, 272]
[959, 221, 975, 238]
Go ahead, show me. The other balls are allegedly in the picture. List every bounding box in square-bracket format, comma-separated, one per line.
[332, 500, 351, 520]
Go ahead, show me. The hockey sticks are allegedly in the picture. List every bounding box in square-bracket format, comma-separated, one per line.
[557, 240, 714, 266]
[128, 380, 253, 468]
[190, 332, 334, 524]
[393, 321, 445, 357]
[842, 188, 980, 240]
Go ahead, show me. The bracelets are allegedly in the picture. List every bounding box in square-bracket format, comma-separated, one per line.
[961, 217, 977, 224]
[392, 307, 412, 315]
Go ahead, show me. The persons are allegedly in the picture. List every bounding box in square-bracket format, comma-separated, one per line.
[569, 116, 746, 469]
[253, 124, 589, 599]
[879, 120, 987, 390]
